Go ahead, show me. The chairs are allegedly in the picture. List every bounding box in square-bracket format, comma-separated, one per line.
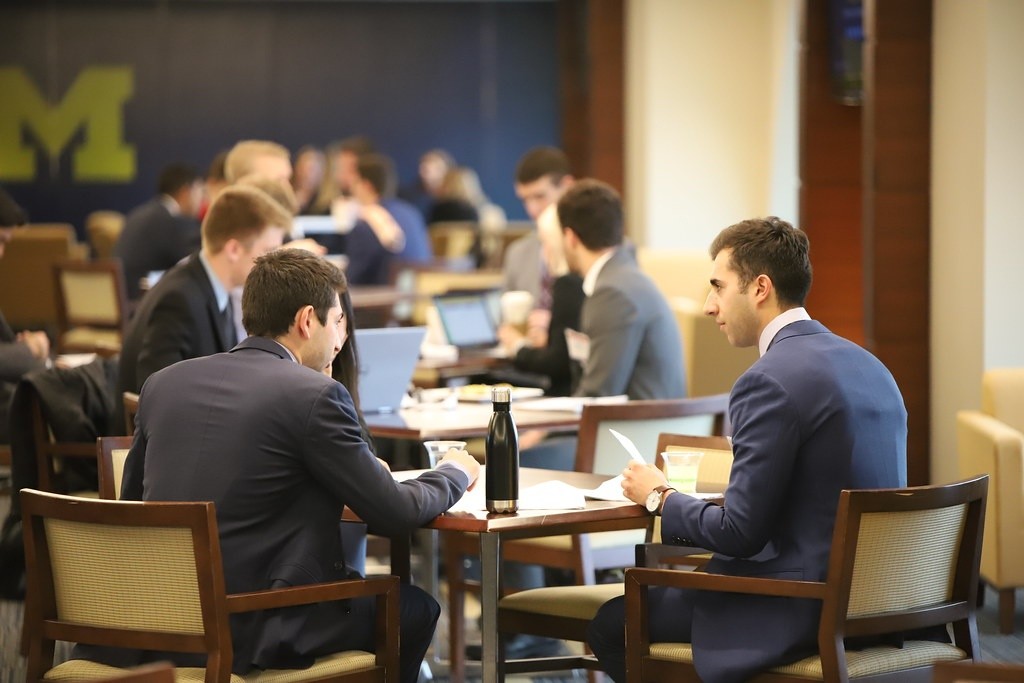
[622, 472, 989, 682]
[0, 210, 744, 679]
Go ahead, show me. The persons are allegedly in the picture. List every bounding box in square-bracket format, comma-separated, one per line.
[504, 145, 577, 306]
[0, 188, 71, 446]
[119, 247, 480, 683]
[117, 138, 504, 322]
[463, 179, 687, 660]
[121, 185, 294, 396]
[498, 202, 584, 397]
[586, 215, 907, 683]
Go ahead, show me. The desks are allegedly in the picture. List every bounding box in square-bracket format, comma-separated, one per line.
[354, 392, 623, 621]
[385, 463, 726, 683]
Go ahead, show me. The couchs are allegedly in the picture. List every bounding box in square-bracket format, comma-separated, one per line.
[958, 366, 1024, 634]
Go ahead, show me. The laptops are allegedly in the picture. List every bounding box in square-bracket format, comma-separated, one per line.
[354, 326, 426, 414]
[433, 286, 504, 347]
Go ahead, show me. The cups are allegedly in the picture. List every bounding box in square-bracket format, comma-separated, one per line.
[423, 442, 467, 471]
[502, 290, 534, 334]
[662, 452, 703, 496]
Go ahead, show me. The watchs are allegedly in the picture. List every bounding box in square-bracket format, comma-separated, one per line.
[645, 484, 678, 516]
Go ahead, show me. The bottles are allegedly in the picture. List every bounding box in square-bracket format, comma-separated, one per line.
[485, 388, 518, 513]
